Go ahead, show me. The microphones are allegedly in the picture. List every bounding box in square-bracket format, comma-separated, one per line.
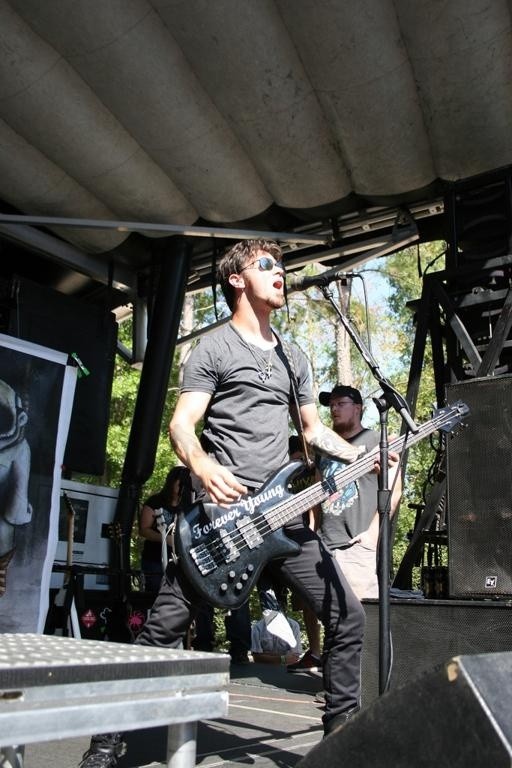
[289, 265, 361, 293]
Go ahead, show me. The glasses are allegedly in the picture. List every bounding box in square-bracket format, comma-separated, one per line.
[239, 258, 285, 272]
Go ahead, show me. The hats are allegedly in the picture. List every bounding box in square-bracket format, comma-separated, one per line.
[319, 386, 362, 406]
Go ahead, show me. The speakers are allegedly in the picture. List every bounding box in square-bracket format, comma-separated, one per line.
[289, 649, 510, 766]
[445, 371, 511, 602]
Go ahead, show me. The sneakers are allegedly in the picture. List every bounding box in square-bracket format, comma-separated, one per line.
[287, 650, 323, 673]
[79, 732, 128, 768]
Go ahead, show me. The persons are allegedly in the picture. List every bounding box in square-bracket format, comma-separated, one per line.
[136, 464, 195, 614]
[286, 381, 401, 672]
[257, 432, 320, 617]
[189, 577, 251, 665]
[77, 236, 399, 766]
[251, 609, 302, 666]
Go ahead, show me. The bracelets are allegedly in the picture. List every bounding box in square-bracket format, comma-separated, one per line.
[356, 442, 369, 462]
[279, 654, 287, 664]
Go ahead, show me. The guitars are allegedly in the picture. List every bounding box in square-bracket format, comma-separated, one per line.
[167, 400, 472, 609]
[107, 522, 125, 570]
